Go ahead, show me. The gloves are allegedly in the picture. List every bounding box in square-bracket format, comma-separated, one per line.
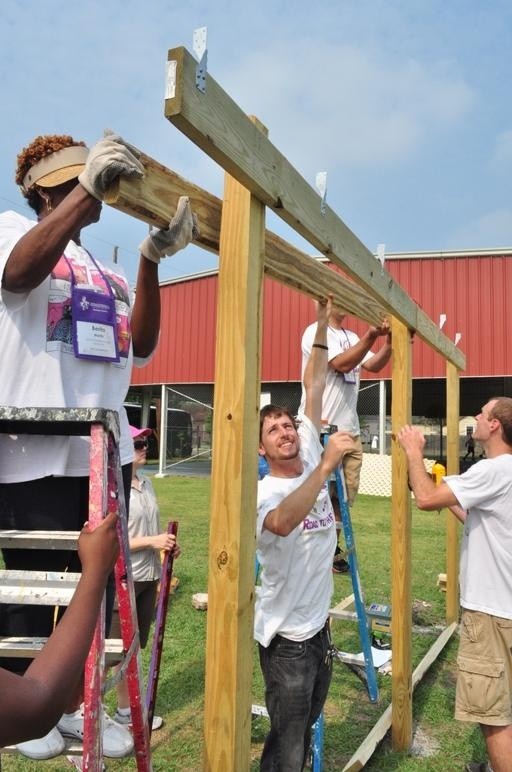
[137, 196, 201, 265]
[77, 136, 147, 203]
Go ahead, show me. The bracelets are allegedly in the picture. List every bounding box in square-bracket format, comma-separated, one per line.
[312, 343, 328, 350]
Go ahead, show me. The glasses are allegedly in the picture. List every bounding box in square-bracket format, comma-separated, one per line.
[133, 440, 150, 451]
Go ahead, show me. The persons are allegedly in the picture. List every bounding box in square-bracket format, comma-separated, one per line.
[1, 506, 124, 748]
[298, 306, 393, 574]
[0, 132, 198, 761]
[396, 396, 511, 771]
[254, 292, 369, 770]
[462, 437, 476, 461]
[113, 424, 183, 734]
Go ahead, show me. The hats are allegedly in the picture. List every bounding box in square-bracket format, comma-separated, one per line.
[21, 144, 92, 196]
[127, 423, 154, 438]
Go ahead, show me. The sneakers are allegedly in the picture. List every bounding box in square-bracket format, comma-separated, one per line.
[332, 543, 349, 576]
[56, 700, 135, 758]
[16, 724, 66, 760]
[116, 706, 164, 734]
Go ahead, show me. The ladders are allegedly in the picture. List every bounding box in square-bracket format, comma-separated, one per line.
[251, 423, 379, 772]
[0, 404, 152, 772]
